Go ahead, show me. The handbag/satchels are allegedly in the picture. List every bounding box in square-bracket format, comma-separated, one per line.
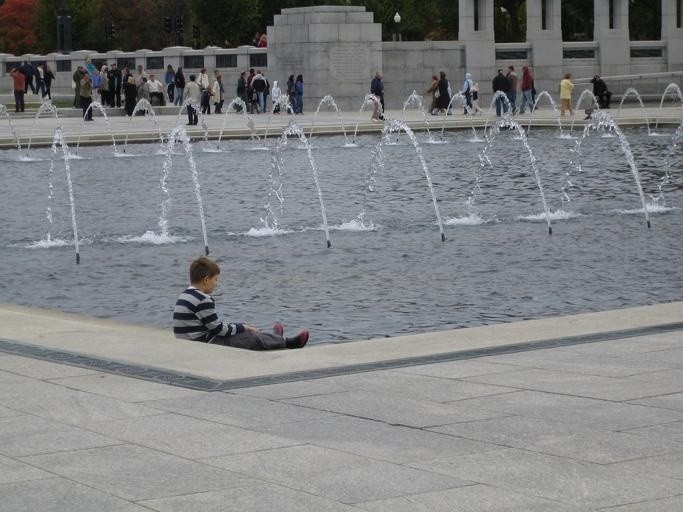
[462, 88, 472, 101]
[435, 88, 440, 97]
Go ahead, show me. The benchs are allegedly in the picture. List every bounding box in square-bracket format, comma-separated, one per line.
[591, 82, 680, 106]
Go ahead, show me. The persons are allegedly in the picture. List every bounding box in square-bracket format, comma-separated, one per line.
[422, 62, 611, 118]
[8, 47, 304, 127]
[370, 71, 387, 122]
[170, 257, 310, 351]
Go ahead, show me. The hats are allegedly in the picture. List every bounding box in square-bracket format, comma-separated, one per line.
[432, 75, 438, 80]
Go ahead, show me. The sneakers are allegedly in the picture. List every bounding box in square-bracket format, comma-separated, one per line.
[272, 323, 283, 335]
[286, 331, 309, 348]
